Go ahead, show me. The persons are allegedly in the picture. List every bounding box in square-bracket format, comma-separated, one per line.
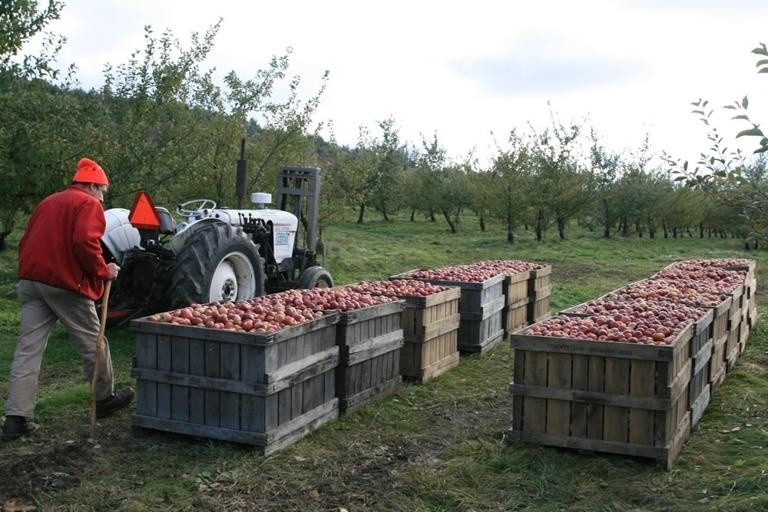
[3, 158, 135, 441]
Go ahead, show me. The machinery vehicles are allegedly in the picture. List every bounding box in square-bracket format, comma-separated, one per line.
[94, 136, 334, 327]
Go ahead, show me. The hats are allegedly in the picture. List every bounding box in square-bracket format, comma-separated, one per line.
[72, 156, 112, 185]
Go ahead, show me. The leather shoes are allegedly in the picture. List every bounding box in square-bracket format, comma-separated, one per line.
[95, 385, 136, 419]
[3, 420, 38, 437]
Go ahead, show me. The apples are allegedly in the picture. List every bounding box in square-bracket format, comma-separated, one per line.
[522, 256, 752, 349]
[145, 257, 545, 347]
